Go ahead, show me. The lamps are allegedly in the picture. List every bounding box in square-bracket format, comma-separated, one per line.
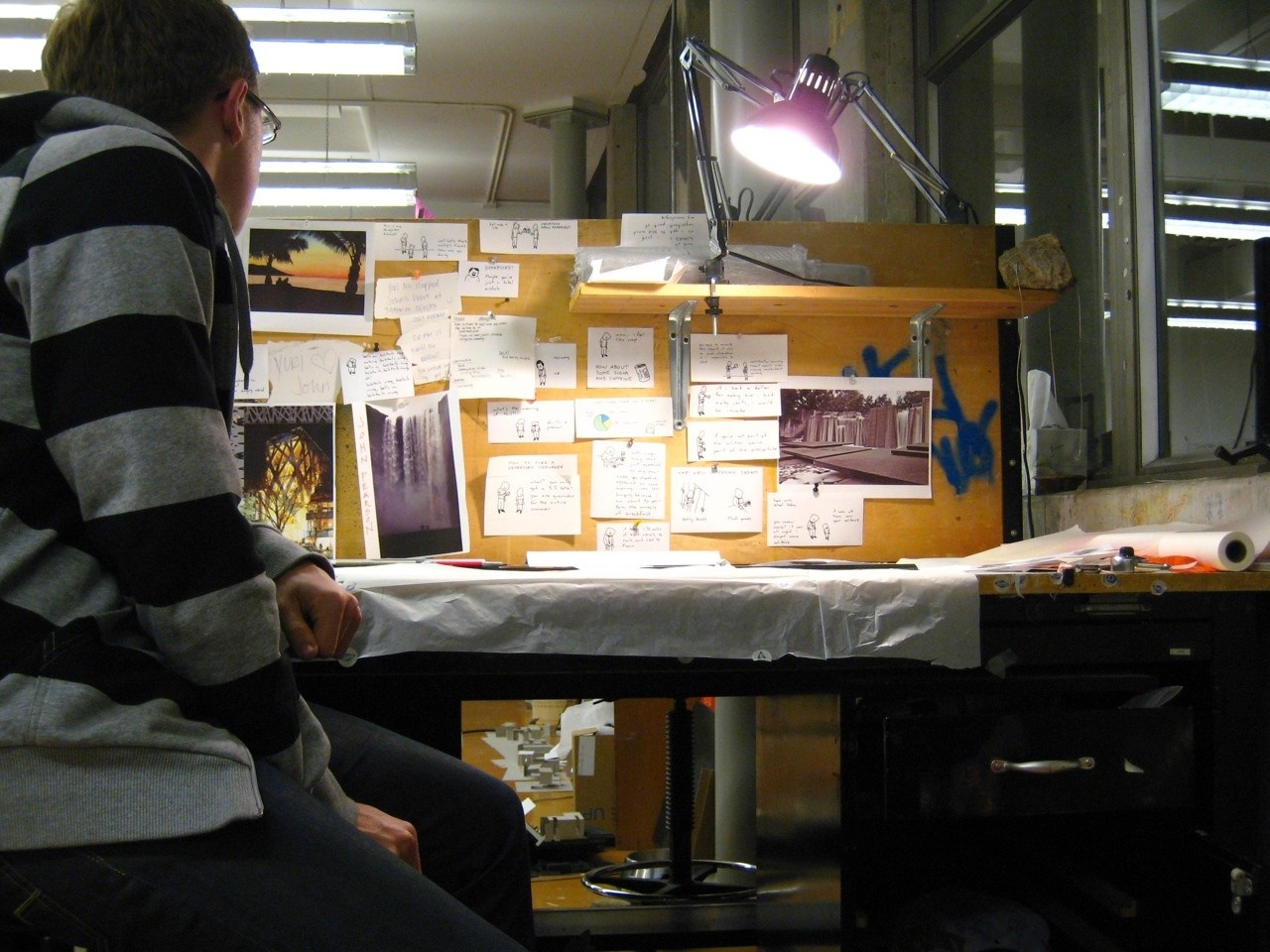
[0, 0, 418, 77]
[680, 32, 969, 303]
[251, 72, 419, 208]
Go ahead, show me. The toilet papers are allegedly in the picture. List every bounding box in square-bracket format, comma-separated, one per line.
[715, 696, 756, 885]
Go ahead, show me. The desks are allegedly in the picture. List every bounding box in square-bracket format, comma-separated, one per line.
[327, 563, 1270, 952]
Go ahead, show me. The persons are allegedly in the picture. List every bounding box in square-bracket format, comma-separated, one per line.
[1, 2, 535, 952]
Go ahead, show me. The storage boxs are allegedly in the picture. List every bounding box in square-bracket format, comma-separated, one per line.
[573, 729, 617, 834]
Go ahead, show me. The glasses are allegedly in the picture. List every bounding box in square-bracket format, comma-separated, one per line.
[214, 86, 281, 145]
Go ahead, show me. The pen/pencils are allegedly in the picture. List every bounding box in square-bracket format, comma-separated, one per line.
[430, 560, 508, 568]
[1137, 563, 1171, 568]
